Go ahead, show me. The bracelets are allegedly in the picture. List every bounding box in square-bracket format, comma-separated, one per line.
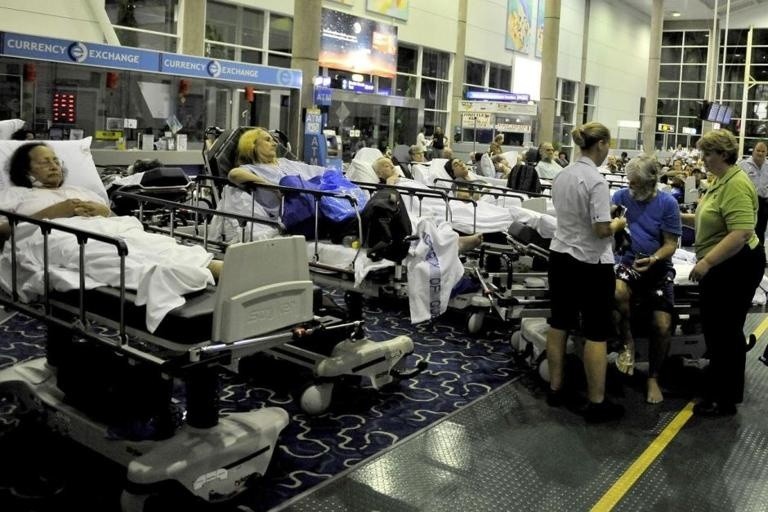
[107, 209, 112, 217]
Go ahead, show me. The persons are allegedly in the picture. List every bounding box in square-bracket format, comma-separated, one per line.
[689, 127, 766, 418]
[0, 142, 224, 279]
[546, 122, 628, 424]
[737, 140, 768, 268]
[227, 127, 484, 256]
[611, 156, 683, 405]
[13, 129, 37, 141]
[228, 128, 717, 231]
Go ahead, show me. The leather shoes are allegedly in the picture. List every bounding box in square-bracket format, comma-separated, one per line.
[545, 389, 569, 405]
[586, 406, 625, 423]
[693, 400, 737, 417]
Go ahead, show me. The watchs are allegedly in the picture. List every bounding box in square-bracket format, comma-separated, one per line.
[653, 255, 660, 262]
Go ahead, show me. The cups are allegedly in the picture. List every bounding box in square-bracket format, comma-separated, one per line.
[177, 134, 187, 151]
[168, 138, 175, 149]
[142, 134, 154, 150]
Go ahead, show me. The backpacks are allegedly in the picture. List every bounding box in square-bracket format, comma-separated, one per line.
[361, 187, 419, 265]
[508, 165, 541, 197]
